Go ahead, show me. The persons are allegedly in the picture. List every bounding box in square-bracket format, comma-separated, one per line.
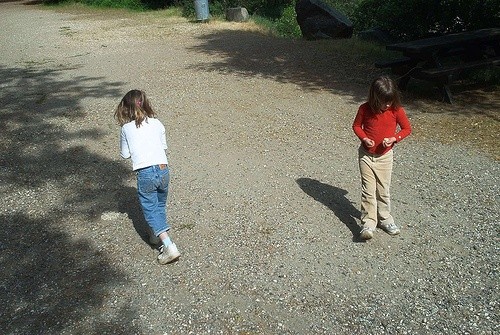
[352, 73, 412, 240]
[113, 89, 181, 264]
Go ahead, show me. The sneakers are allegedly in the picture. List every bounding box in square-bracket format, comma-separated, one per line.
[149, 228, 161, 244]
[359, 226, 374, 240]
[156, 243, 181, 265]
[377, 221, 400, 235]
